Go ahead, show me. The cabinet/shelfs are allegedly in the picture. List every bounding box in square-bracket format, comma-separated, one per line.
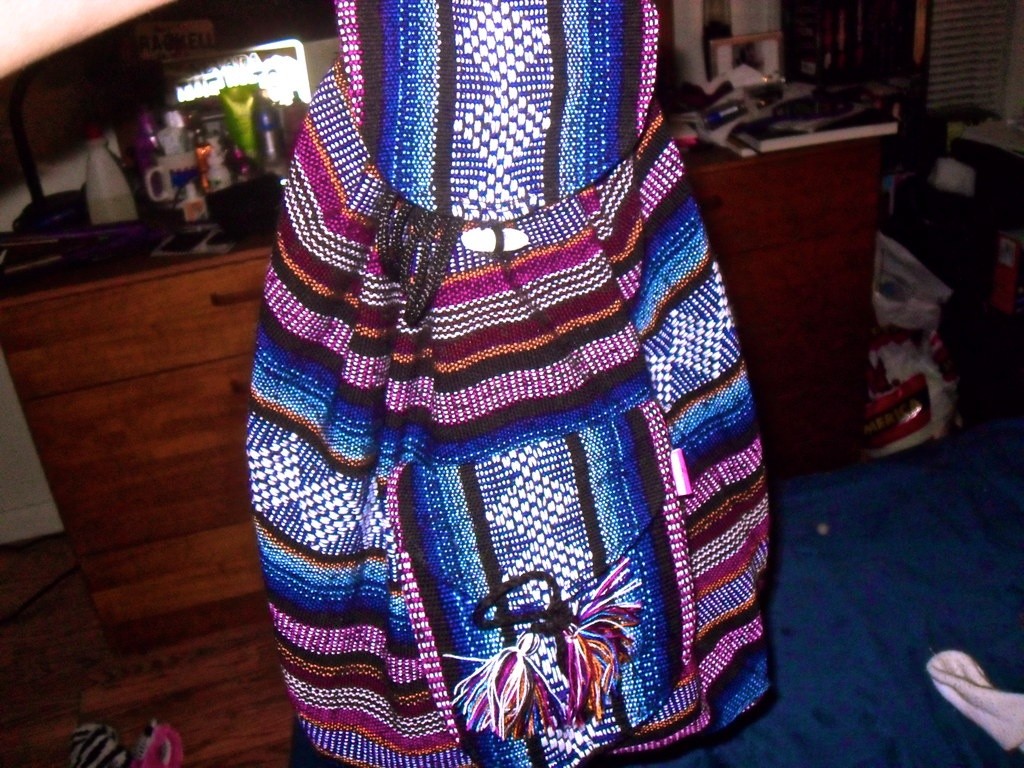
[0, 106, 901, 674]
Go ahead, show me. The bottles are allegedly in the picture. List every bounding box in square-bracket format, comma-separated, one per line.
[82, 122, 141, 227]
[255, 96, 285, 164]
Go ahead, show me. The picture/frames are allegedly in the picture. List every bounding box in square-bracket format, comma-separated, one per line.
[711, 31, 784, 86]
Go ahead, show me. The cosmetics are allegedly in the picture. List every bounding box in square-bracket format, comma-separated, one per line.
[84, 81, 308, 228]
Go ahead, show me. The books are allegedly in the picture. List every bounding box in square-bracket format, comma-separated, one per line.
[734, 106, 900, 155]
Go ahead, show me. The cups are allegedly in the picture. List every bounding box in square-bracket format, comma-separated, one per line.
[141, 149, 199, 203]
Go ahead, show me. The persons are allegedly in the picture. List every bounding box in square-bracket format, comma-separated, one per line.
[0, 0, 176, 84]
